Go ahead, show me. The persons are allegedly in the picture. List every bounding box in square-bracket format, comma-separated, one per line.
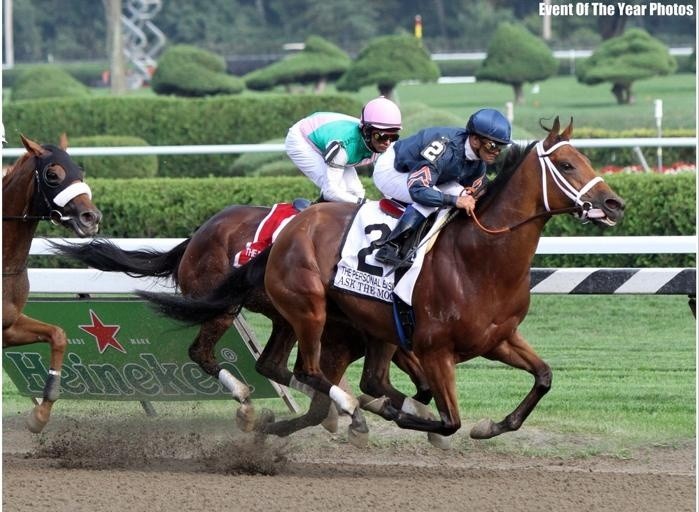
[372, 109, 512, 266]
[285, 97, 402, 207]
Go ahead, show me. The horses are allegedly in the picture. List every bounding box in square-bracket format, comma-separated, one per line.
[1, 132, 104, 434]
[37, 204, 435, 433]
[131, 115, 627, 449]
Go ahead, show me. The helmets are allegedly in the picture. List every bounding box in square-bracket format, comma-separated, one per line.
[465, 109, 510, 144]
[360, 95, 402, 132]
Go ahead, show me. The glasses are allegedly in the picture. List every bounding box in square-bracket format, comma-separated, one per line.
[373, 130, 399, 142]
[479, 137, 507, 154]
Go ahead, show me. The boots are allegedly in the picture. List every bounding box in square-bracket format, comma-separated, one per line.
[375, 203, 427, 267]
